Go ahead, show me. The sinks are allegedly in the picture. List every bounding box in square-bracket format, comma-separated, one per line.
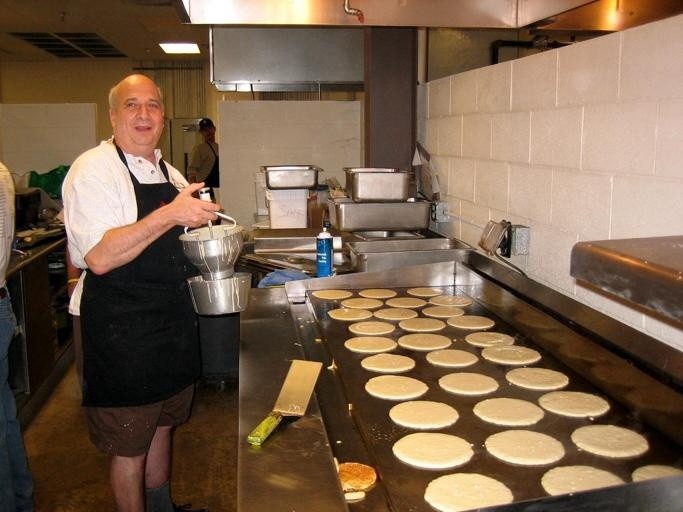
[345, 232, 472, 273]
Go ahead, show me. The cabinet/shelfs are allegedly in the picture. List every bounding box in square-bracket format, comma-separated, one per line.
[4, 218, 75, 432]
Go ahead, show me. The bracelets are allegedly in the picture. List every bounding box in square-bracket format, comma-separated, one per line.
[65, 278, 80, 284]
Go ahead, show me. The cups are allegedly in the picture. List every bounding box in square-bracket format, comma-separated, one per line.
[178, 225, 244, 280]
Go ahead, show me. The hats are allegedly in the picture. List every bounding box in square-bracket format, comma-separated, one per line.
[199, 119, 213, 130]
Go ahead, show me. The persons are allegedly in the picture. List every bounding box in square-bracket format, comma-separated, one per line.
[186, 118, 218, 197]
[1, 163, 35, 512]
[67, 239, 84, 300]
[61, 74, 224, 512]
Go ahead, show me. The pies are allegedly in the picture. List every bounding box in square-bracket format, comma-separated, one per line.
[334, 455, 377, 499]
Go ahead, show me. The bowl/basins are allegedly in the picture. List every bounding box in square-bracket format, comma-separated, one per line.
[185, 272, 252, 316]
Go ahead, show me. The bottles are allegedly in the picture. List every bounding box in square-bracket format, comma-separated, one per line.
[316, 227, 333, 278]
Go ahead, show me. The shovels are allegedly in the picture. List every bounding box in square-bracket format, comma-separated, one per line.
[246, 359, 323, 445]
